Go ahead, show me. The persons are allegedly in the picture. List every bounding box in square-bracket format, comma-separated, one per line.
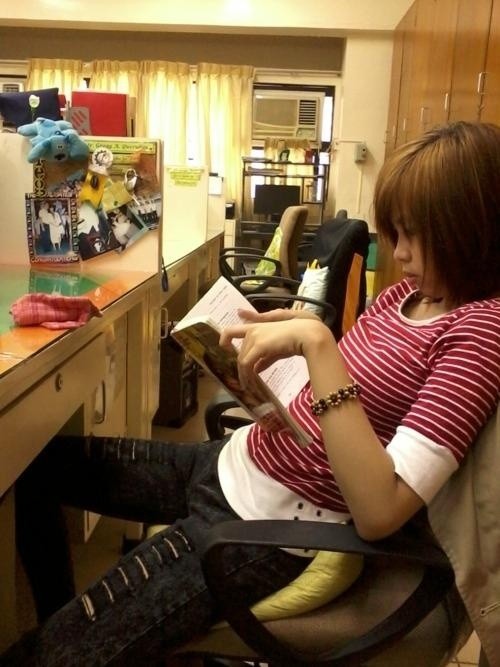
[32, 200, 71, 255]
[128, 193, 158, 225]
[0, 118, 499, 665]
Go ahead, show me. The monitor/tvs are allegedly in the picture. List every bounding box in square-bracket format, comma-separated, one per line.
[255, 184, 302, 222]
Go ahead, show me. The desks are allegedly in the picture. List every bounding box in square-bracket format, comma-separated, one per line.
[0, 226, 228, 655]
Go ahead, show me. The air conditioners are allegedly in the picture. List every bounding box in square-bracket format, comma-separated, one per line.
[251, 89, 325, 149]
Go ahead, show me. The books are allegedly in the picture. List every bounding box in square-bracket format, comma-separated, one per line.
[170, 274, 313, 449]
[73, 91, 132, 137]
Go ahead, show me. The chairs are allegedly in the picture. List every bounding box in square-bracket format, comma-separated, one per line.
[199, 369, 500, 666]
[227, 219, 371, 326]
[221, 206, 308, 292]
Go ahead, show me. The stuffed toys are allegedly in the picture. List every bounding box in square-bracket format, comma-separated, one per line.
[17, 116, 90, 165]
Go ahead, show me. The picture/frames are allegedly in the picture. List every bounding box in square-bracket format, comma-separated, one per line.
[24, 192, 80, 262]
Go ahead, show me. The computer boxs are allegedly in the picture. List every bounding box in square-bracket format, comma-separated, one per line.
[152, 326, 199, 429]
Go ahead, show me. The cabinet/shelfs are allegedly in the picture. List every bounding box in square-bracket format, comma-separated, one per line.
[244, 157, 328, 223]
[384, 1, 500, 299]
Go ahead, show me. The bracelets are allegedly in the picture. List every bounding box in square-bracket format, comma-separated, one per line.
[310, 381, 363, 415]
